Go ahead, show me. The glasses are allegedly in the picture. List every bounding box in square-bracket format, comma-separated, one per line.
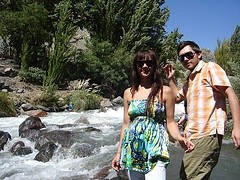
[178, 52, 196, 62]
[135, 60, 154, 67]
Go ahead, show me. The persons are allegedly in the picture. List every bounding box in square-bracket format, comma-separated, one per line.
[112, 49, 195, 180]
[163, 40, 240, 180]
